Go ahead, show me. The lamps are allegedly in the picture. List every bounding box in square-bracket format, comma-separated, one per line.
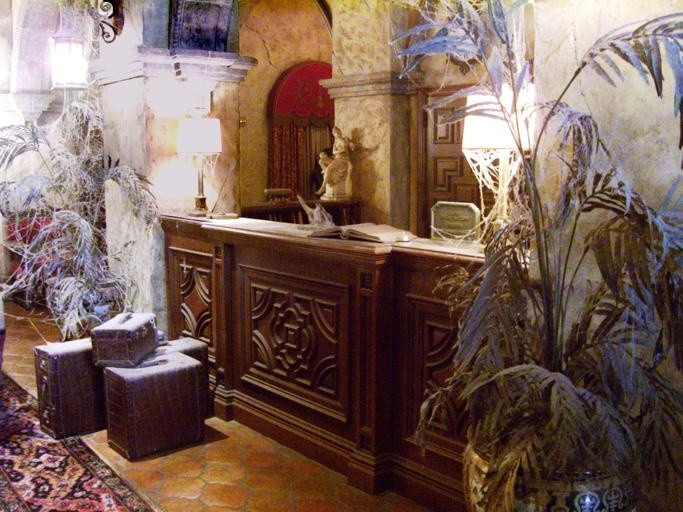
[175, 116, 223, 218]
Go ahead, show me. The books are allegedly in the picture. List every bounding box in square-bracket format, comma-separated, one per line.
[307, 222, 417, 244]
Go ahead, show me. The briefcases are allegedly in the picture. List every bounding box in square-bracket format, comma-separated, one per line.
[34, 314, 210, 462]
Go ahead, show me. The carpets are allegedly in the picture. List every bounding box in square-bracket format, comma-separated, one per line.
[0, 371, 163, 512]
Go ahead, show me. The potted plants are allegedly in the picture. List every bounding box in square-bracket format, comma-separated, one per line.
[384, 0, 683, 512]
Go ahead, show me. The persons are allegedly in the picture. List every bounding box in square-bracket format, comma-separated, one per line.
[313, 126, 354, 198]
[317, 152, 339, 172]
